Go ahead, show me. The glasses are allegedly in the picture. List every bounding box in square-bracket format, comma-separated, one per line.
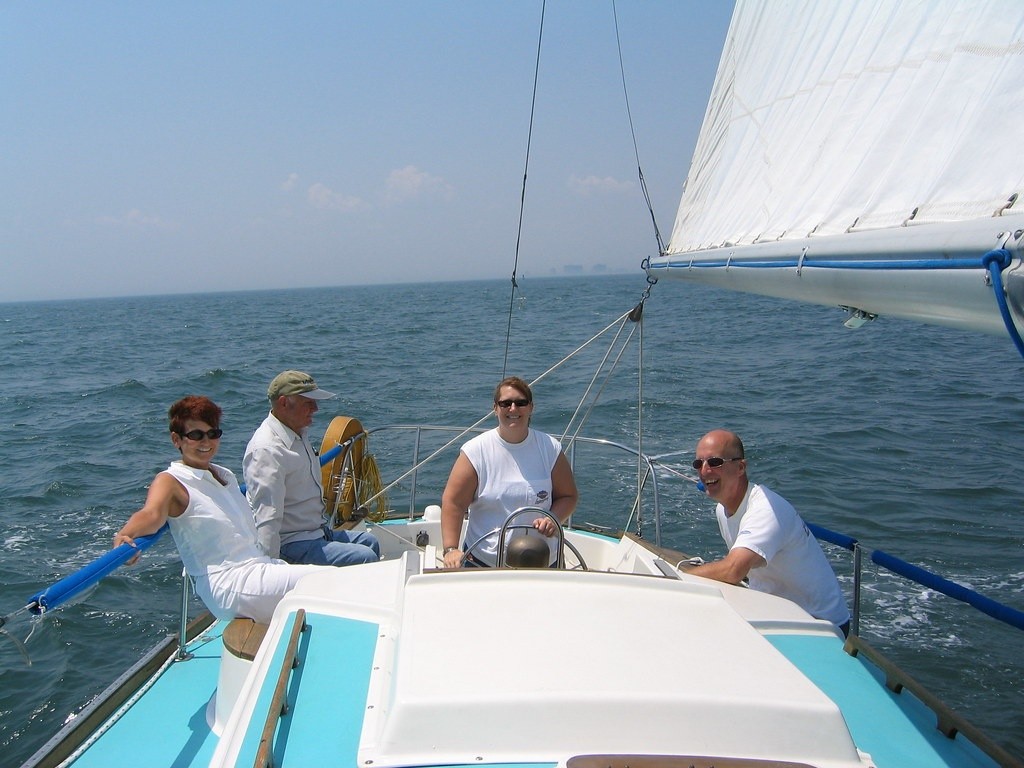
[179, 429, 223, 441]
[496, 398, 531, 408]
[693, 457, 744, 469]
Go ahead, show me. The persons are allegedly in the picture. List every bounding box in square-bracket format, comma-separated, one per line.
[442, 376, 579, 568]
[113, 397, 340, 625]
[686, 429, 852, 640]
[242, 370, 380, 568]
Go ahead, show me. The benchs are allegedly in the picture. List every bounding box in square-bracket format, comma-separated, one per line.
[214, 617, 268, 728]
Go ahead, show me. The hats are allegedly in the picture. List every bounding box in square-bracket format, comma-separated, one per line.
[268, 370, 336, 401]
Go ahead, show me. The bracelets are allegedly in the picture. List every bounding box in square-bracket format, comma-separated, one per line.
[442, 546, 458, 557]
